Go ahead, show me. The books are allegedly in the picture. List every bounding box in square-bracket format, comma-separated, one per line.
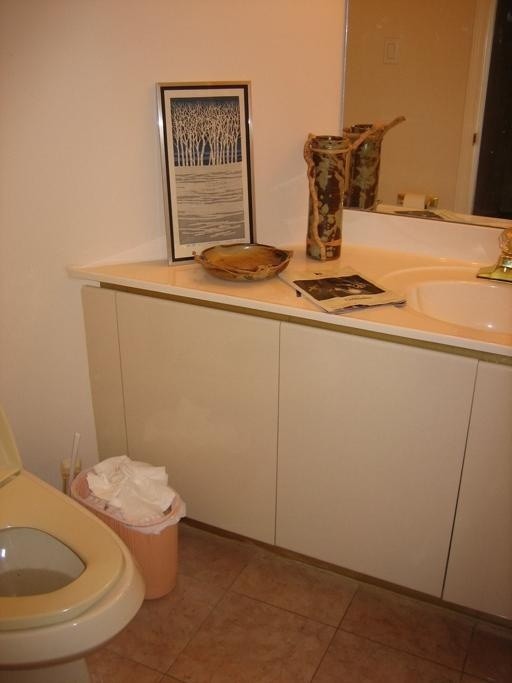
[278, 264, 406, 315]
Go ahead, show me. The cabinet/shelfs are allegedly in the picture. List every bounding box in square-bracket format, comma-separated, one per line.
[82, 271, 512, 636]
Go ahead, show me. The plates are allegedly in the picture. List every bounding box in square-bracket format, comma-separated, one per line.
[191, 240, 296, 281]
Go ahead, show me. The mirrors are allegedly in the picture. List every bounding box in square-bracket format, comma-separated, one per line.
[337, 1, 512, 228]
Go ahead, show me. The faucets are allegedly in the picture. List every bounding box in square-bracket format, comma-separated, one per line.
[476, 227, 512, 282]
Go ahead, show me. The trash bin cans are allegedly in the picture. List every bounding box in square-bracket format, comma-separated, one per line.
[70, 466, 181, 600]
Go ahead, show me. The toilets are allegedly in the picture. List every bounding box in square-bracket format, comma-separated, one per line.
[0, 406, 146, 683]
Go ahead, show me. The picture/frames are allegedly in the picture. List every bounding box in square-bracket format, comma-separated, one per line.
[381, 36, 400, 65]
[154, 76, 258, 266]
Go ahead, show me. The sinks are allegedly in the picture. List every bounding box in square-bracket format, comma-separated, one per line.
[383, 265, 512, 335]
